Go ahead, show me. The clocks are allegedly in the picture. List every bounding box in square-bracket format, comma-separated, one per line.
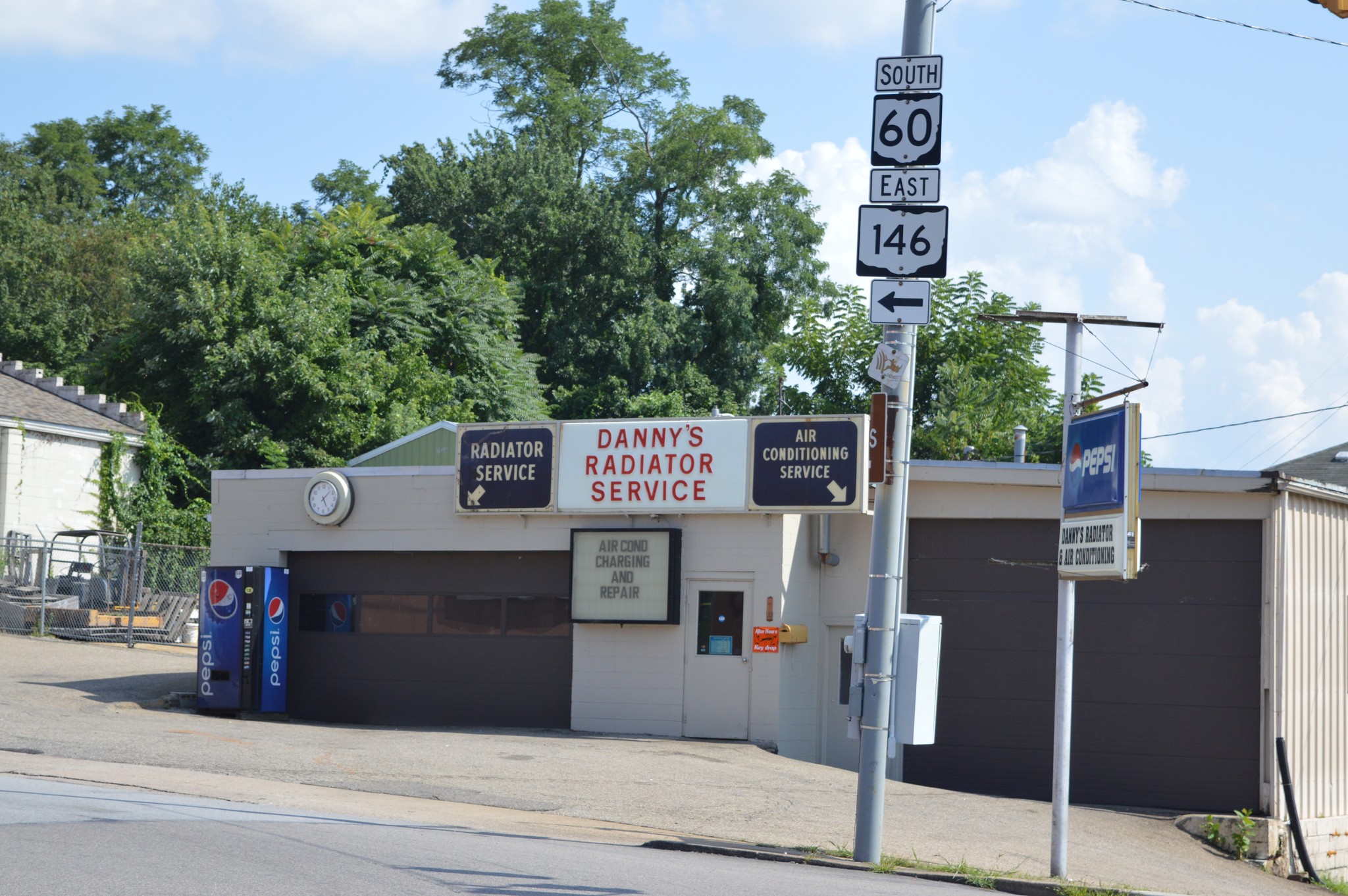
[303, 469, 355, 526]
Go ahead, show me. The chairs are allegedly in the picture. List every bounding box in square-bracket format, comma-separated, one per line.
[71, 562, 93, 582]
